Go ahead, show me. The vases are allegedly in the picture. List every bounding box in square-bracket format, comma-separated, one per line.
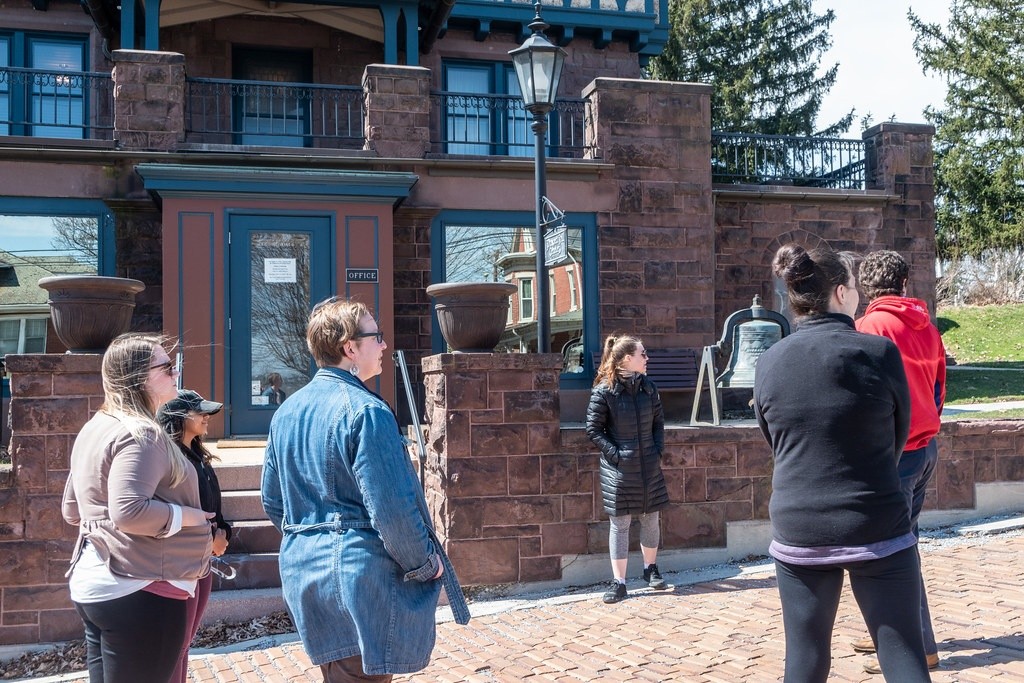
[38, 275, 146, 354]
[425, 282, 519, 353]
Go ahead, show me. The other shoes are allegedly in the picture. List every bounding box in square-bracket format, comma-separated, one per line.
[850, 639, 876, 651]
[862, 653, 939, 673]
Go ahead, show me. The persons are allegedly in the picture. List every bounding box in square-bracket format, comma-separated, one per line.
[262, 373, 286, 405]
[753, 243, 932, 683]
[260, 303, 470, 683]
[851, 250, 946, 674]
[586, 334, 670, 602]
[60, 333, 232, 683]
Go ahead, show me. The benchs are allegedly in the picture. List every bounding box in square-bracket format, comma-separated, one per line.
[589, 347, 710, 420]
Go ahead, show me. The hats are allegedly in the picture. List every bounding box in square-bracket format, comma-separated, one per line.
[157, 389, 223, 423]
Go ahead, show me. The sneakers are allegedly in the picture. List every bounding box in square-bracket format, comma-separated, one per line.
[603, 579, 627, 602]
[643, 564, 666, 588]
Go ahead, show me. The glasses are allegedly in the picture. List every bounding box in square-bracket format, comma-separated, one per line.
[351, 331, 383, 343]
[143, 360, 173, 376]
[631, 350, 648, 359]
